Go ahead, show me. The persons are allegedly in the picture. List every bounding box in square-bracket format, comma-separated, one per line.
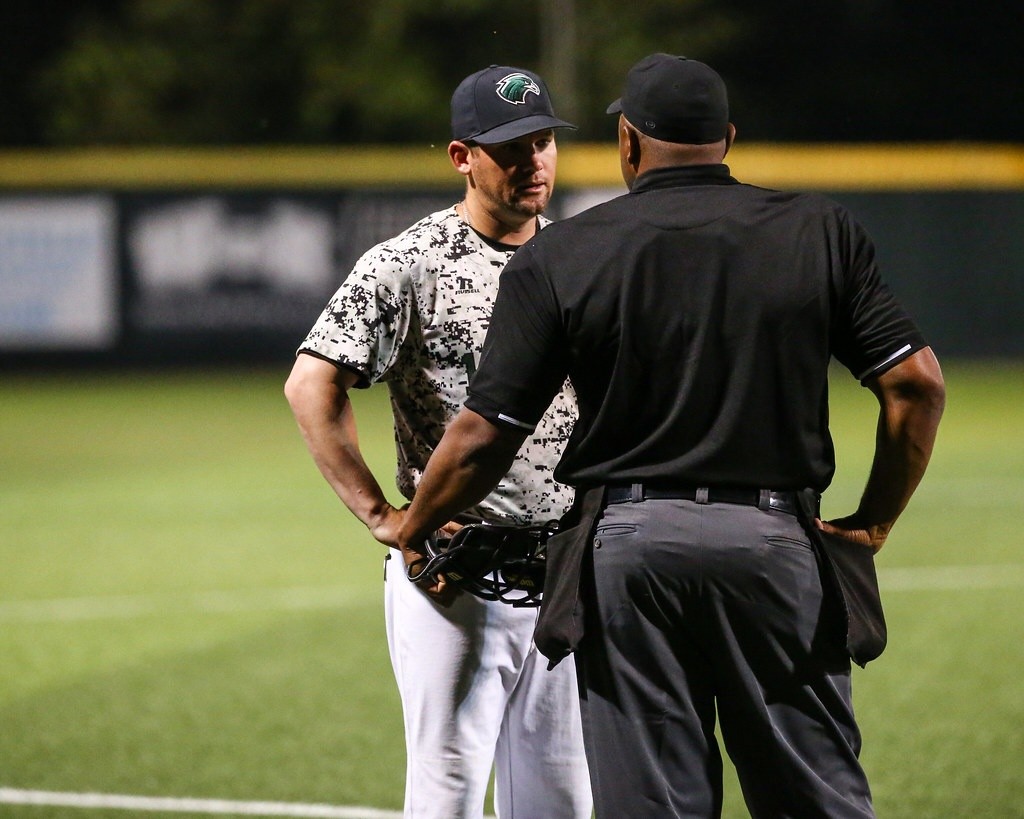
[283, 66, 593, 819]
[397, 53, 944, 819]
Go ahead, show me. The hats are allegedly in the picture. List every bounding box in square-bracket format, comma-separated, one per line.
[451, 64, 577, 146]
[605, 53, 728, 145]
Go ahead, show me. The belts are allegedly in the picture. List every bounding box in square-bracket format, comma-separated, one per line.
[595, 481, 800, 516]
